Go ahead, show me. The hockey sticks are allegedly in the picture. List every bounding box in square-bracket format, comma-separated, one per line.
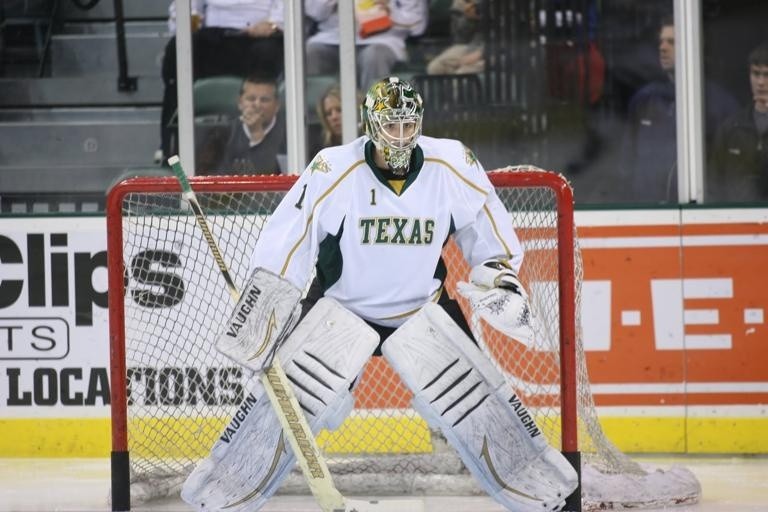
[167, 155, 425, 512]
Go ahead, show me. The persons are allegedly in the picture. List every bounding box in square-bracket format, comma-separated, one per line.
[161, 0, 768, 205]
[177, 75, 579, 512]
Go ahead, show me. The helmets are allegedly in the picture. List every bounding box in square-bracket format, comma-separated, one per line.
[362, 76, 424, 176]
